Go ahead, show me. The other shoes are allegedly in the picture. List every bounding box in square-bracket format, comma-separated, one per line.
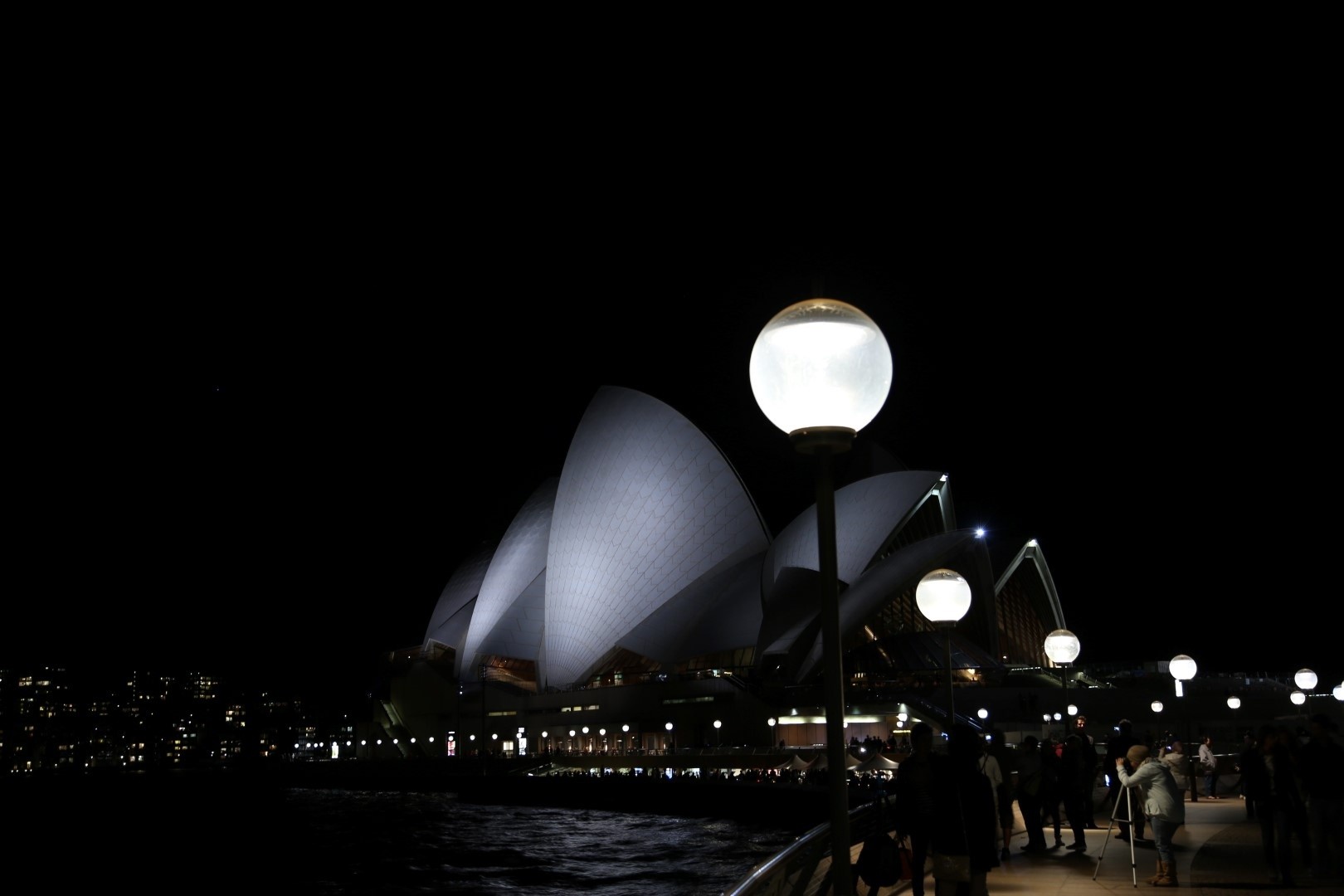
[1115, 835, 1129, 839]
[1020, 845, 1028, 850]
[1056, 840, 1065, 848]
[1001, 847, 1010, 861]
[1134, 836, 1146, 842]
[1066, 843, 1087, 849]
[1021, 850, 1029, 856]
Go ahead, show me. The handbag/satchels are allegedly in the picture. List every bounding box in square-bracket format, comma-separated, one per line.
[897, 838, 913, 880]
[932, 855, 971, 883]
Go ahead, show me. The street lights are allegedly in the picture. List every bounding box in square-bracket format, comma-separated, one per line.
[1289, 692, 1306, 718]
[1149, 701, 1164, 740]
[1060, 689, 1090, 703]
[1168, 655, 1199, 731]
[1227, 696, 1241, 739]
[746, 297, 892, 896]
[1294, 669, 1317, 713]
[1045, 628, 1081, 728]
[912, 568, 975, 736]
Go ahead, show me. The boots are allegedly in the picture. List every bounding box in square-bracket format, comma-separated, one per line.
[1146, 860, 1164, 884]
[1151, 861, 1178, 886]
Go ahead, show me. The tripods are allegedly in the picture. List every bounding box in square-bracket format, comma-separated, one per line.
[1091, 782, 1159, 889]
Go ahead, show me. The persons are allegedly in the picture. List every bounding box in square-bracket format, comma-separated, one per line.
[895, 705, 1344, 896]
[1018, 691, 1039, 712]
[478, 739, 676, 761]
[857, 672, 942, 692]
[1224, 682, 1297, 696]
[850, 729, 911, 754]
[546, 766, 879, 792]
